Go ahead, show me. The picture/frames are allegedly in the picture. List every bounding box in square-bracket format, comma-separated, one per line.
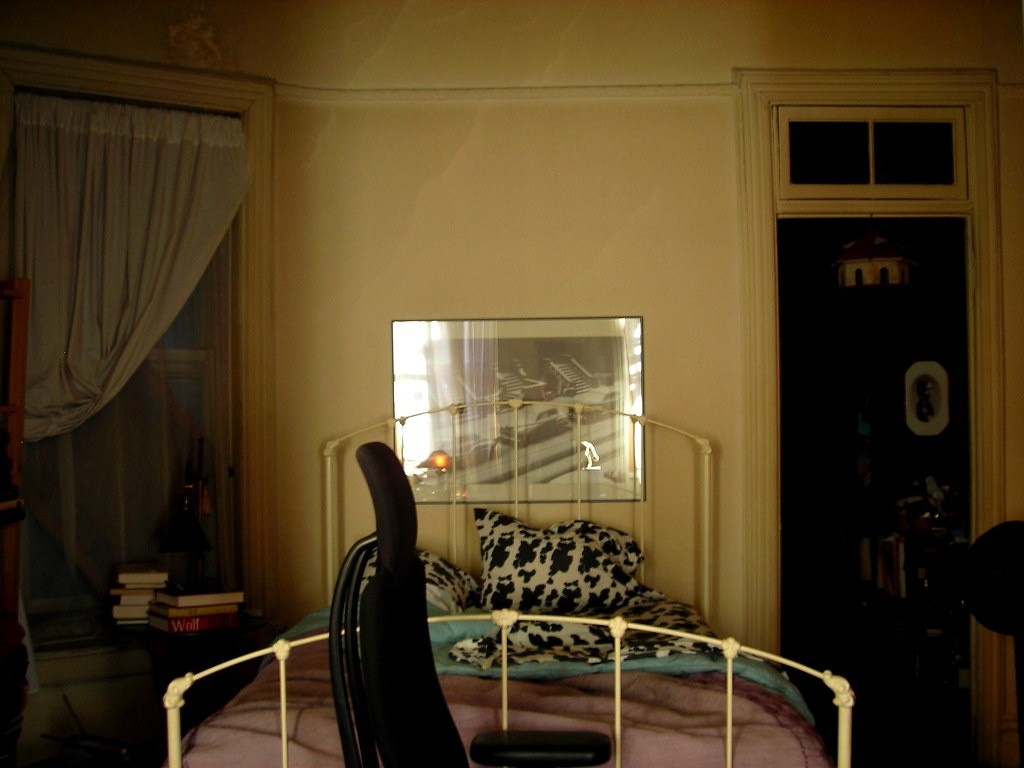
[393, 315, 646, 506]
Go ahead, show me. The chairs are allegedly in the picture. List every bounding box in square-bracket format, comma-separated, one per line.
[329, 441, 613, 768]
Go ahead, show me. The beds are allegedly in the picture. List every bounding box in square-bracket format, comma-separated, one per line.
[165, 400, 855, 768]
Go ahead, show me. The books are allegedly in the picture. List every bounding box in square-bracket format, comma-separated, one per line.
[111, 562, 244, 631]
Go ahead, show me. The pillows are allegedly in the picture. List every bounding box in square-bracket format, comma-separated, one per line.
[474, 508, 668, 623]
[360, 544, 480, 616]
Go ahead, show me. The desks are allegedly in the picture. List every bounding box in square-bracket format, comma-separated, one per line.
[103, 608, 269, 738]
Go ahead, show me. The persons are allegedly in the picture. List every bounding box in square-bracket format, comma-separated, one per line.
[916, 378, 937, 422]
